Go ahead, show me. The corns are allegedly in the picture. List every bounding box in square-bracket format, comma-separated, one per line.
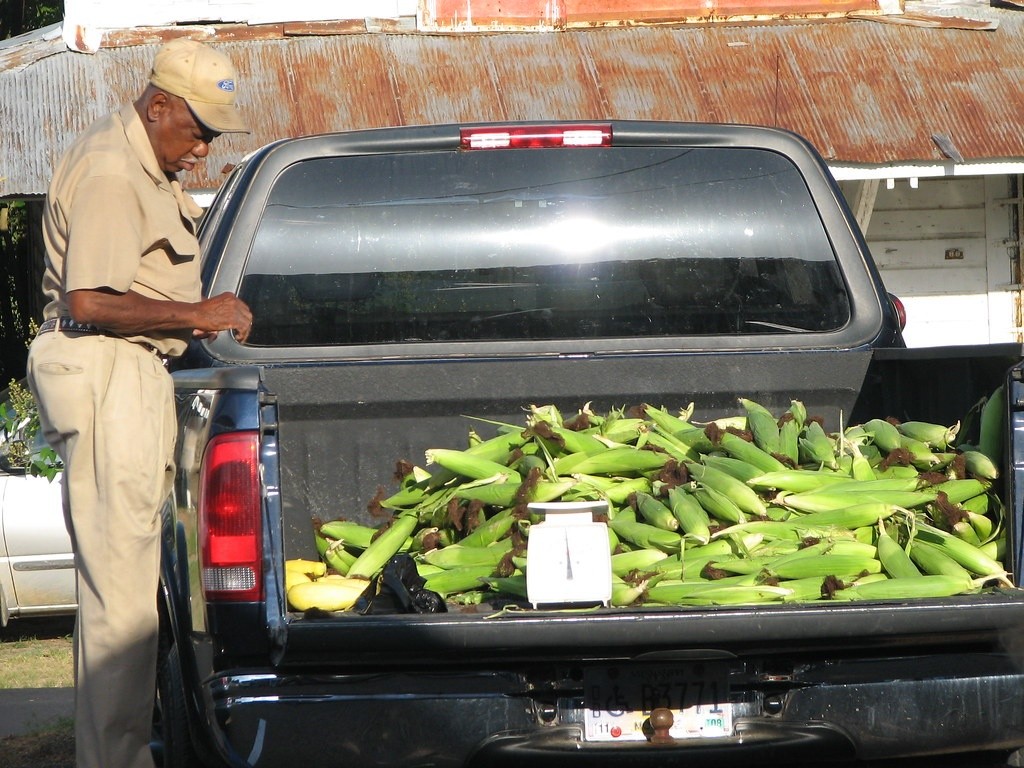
[313, 396, 1015, 607]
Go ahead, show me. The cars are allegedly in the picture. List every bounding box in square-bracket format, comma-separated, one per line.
[0, 377, 78, 640]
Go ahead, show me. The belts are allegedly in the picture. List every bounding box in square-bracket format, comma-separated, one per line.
[38, 317, 169, 370]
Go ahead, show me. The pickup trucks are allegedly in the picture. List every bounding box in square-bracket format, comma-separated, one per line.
[154, 120, 1024, 768]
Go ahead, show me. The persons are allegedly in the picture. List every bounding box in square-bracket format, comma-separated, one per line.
[25, 37, 254, 768]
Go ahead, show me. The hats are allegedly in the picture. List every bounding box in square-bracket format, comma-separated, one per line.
[147, 38, 251, 134]
